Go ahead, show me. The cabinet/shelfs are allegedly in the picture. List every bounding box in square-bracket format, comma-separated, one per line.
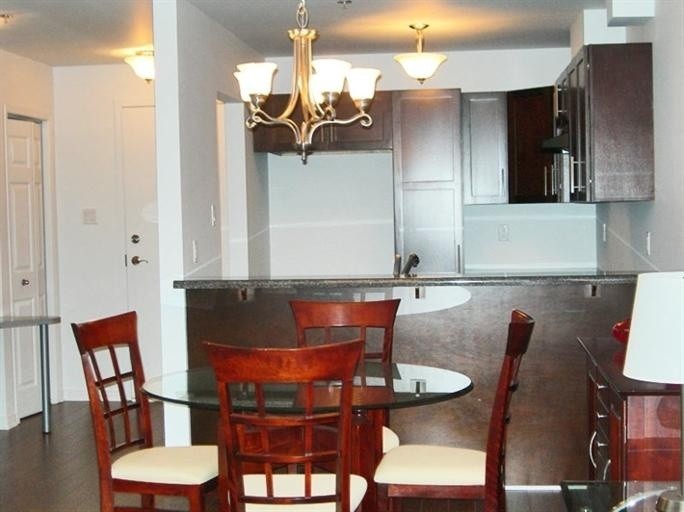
[461, 42, 655, 205]
[578, 336, 684, 512]
[252, 91, 393, 151]
[393, 89, 461, 275]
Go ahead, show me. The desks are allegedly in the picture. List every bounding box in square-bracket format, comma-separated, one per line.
[0, 315, 60, 434]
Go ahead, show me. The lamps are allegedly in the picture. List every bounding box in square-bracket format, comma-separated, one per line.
[393, 24, 448, 84]
[124, 53, 154, 83]
[622, 271, 684, 512]
[233, 1, 381, 163]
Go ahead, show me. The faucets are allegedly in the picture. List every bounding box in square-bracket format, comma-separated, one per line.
[394, 253, 420, 277]
